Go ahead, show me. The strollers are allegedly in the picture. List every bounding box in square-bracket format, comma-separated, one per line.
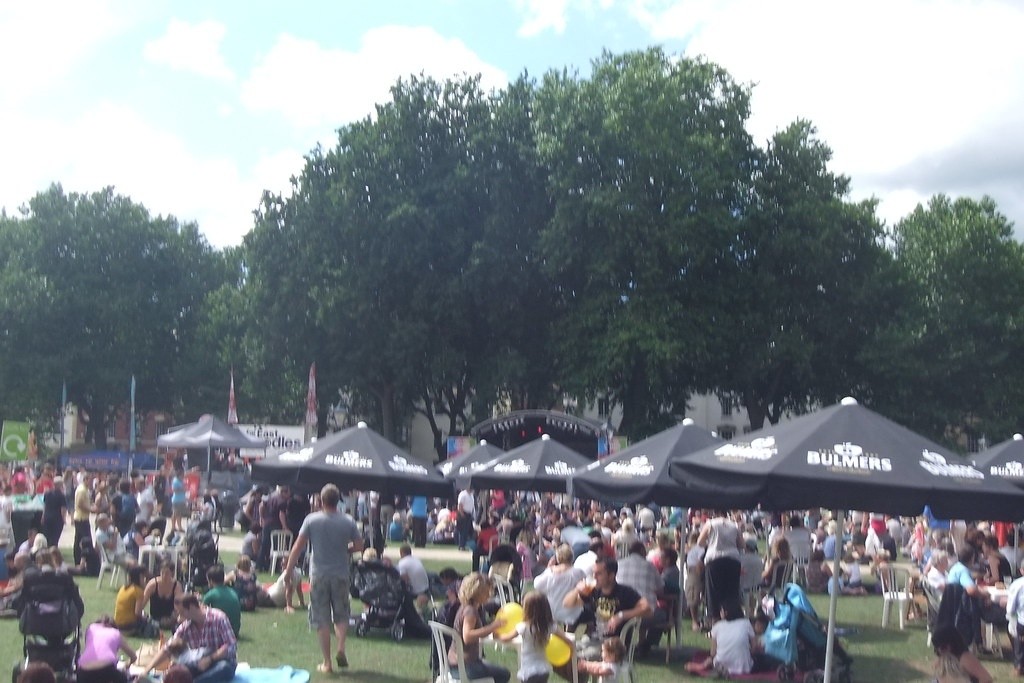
[144, 518, 167, 545]
[350, 559, 432, 642]
[12, 571, 84, 683]
[189, 520, 223, 587]
[763, 582, 856, 683]
[483, 544, 523, 618]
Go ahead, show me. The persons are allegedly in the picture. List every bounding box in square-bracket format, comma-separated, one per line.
[284, 483, 363, 674]
[0, 447, 1024, 683]
[74, 477, 92, 565]
[399, 542, 429, 595]
[698, 509, 750, 638]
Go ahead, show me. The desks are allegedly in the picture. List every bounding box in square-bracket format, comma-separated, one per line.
[137, 546, 193, 582]
[479, 631, 578, 682]
[977, 585, 1010, 659]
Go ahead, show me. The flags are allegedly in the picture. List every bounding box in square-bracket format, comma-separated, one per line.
[306, 363, 317, 422]
[227, 365, 237, 423]
[131, 376, 135, 452]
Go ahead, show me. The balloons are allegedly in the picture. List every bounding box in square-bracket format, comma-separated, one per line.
[547, 633, 571, 667]
[496, 602, 522, 635]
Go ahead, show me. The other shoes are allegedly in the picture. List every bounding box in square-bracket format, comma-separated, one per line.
[459, 545, 469, 551]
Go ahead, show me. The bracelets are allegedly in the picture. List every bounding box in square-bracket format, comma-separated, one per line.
[209, 654, 215, 663]
[618, 611, 624, 620]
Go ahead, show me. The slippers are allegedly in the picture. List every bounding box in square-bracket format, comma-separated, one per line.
[316, 663, 333, 673]
[336, 651, 348, 667]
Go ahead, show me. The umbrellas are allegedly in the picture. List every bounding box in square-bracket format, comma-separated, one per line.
[456, 432, 593, 560]
[670, 397, 1024, 683]
[435, 441, 510, 519]
[568, 418, 752, 651]
[250, 424, 449, 518]
[967, 433, 1024, 577]
[159, 416, 267, 482]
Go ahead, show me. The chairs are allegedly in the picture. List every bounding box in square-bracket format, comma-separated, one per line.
[301, 538, 312, 574]
[270, 529, 294, 577]
[494, 574, 528, 653]
[428, 621, 495, 683]
[96, 542, 131, 590]
[614, 541, 633, 559]
[789, 540, 812, 586]
[878, 562, 917, 630]
[921, 576, 941, 647]
[766, 559, 794, 596]
[589, 616, 641, 682]
[638, 593, 677, 666]
